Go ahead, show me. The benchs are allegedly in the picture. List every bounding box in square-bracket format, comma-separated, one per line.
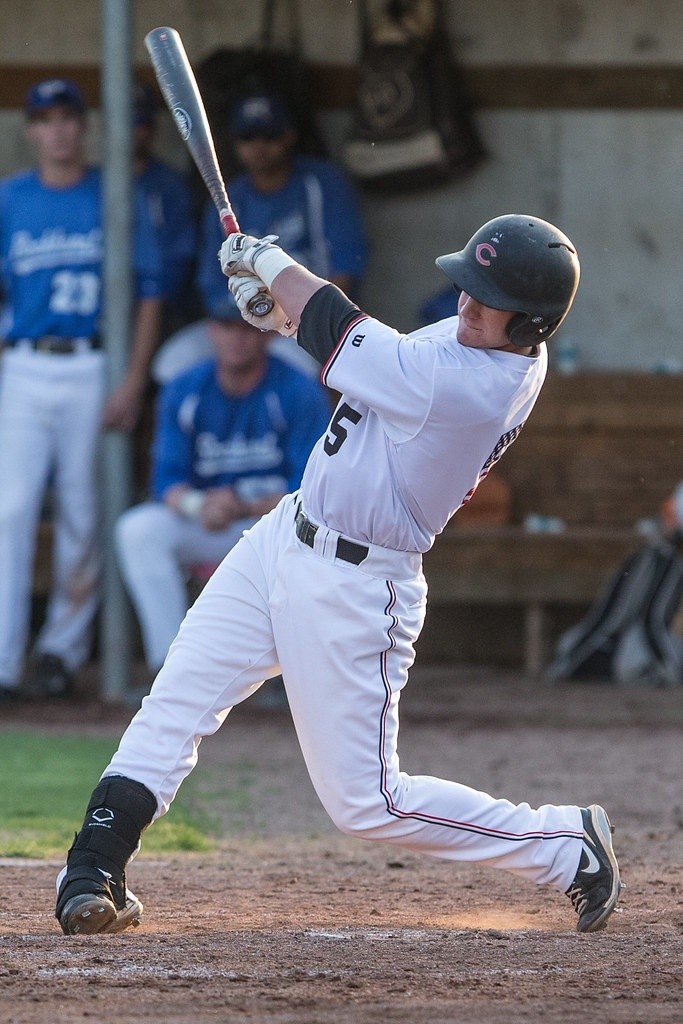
[31, 371, 683, 680]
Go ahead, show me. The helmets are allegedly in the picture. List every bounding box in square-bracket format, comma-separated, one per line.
[436, 214, 581, 348]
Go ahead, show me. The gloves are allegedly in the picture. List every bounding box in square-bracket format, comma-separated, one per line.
[227, 270, 298, 338]
[218, 232, 281, 275]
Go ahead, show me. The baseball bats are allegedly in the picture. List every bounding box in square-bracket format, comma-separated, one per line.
[142, 25, 275, 317]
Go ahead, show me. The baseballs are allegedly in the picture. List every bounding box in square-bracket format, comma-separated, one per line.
[637, 518, 656, 534]
[525, 513, 566, 534]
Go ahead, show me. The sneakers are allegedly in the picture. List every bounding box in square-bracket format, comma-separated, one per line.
[565, 804, 626, 933]
[55, 854, 144, 936]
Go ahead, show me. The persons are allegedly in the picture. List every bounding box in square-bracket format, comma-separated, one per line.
[1, 79, 373, 702]
[54, 212, 619, 935]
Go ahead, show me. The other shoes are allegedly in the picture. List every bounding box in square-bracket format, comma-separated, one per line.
[32, 655, 77, 698]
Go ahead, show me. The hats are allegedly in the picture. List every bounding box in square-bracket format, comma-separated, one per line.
[210, 283, 243, 324]
[227, 98, 280, 140]
[25, 81, 87, 119]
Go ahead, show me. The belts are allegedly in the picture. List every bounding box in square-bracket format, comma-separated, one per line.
[294, 494, 369, 567]
[11, 334, 104, 353]
